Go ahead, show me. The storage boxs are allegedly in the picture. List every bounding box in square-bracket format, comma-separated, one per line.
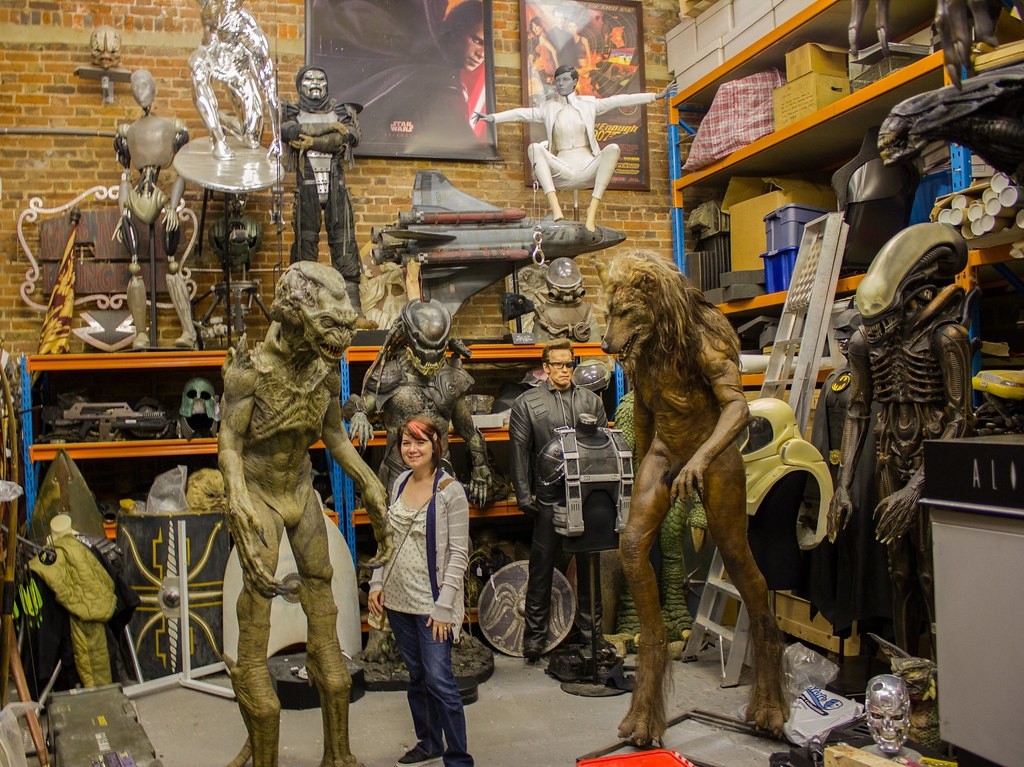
[772, 71, 850, 133]
[759, 246, 797, 294]
[45, 681, 163, 767]
[762, 203, 830, 251]
[721, 173, 837, 272]
[783, 41, 850, 82]
[662, 1, 818, 94]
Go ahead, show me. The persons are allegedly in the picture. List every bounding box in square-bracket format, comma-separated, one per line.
[279, 62, 378, 330]
[509, 340, 617, 664]
[368, 416, 474, 767]
[113, 66, 200, 348]
[536, 415, 635, 555]
[528, 5, 604, 99]
[188, 0, 283, 162]
[312, 0, 486, 150]
[468, 64, 678, 233]
[807, 308, 862, 481]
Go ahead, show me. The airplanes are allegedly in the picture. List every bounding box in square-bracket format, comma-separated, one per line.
[371, 168, 627, 328]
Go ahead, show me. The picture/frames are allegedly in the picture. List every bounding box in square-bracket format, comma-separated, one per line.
[518, 0, 650, 191]
[303, 0, 500, 162]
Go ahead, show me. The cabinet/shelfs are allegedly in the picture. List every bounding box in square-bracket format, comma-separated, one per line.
[20, 340, 625, 633]
[664, 1, 1024, 412]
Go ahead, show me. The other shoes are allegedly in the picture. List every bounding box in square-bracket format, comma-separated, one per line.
[396, 749, 444, 767]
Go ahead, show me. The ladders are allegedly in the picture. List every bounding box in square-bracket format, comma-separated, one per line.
[683, 211, 849, 688]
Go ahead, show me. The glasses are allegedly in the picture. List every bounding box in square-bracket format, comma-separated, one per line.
[545, 361, 575, 369]
